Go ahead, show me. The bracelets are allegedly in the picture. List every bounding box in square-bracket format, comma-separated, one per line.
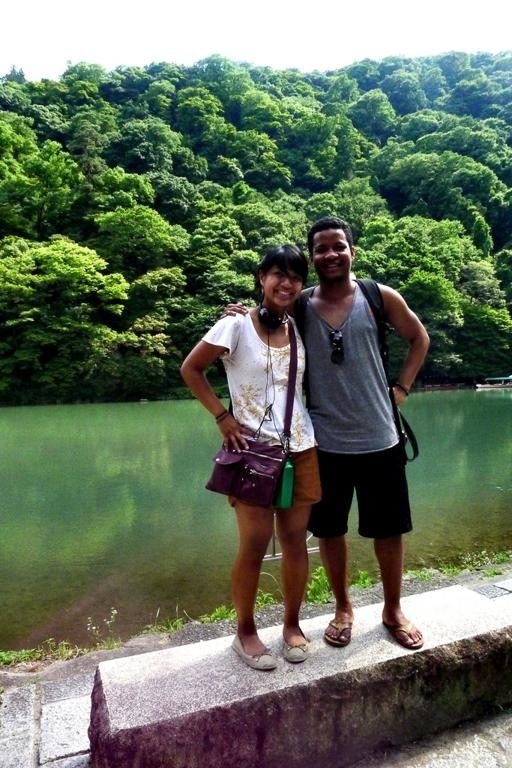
[216, 410, 229, 424]
[392, 382, 410, 398]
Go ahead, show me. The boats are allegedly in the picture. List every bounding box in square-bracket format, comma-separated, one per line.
[477, 374, 512, 387]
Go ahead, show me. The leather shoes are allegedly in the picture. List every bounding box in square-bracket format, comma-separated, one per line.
[283, 627, 308, 663]
[232, 636, 277, 670]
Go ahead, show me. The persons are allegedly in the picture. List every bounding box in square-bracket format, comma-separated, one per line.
[219, 216, 431, 650]
[178, 243, 324, 670]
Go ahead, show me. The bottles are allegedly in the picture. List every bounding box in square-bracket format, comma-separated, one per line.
[276, 457, 295, 509]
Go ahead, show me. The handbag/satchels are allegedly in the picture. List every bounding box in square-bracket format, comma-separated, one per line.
[205, 438, 287, 507]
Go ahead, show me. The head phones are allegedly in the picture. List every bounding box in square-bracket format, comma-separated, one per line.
[257, 301, 289, 333]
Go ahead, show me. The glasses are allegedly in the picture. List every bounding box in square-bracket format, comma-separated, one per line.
[329, 330, 344, 364]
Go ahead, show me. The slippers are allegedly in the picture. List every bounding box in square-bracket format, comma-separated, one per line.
[383, 621, 424, 649]
[325, 621, 352, 646]
